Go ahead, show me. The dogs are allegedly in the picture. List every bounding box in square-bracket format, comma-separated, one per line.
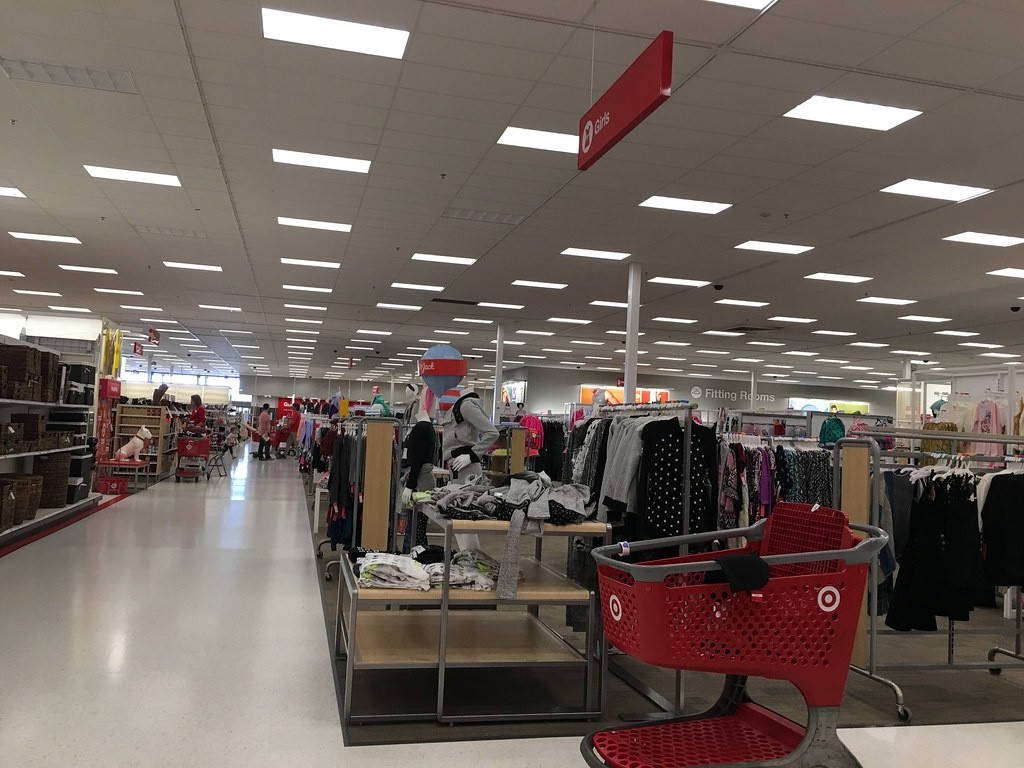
[110, 424, 152, 462]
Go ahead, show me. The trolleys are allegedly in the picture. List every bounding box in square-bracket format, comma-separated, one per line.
[580, 499, 892, 768]
[175, 428, 215, 482]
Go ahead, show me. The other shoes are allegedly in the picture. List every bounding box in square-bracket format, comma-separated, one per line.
[295, 457, 298, 460]
[259, 458, 266, 461]
[280, 454, 286, 459]
[232, 457, 237, 459]
[267, 457, 275, 460]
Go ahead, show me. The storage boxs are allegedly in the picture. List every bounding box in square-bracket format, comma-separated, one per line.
[97, 477, 129, 496]
[67, 483, 91, 504]
[70, 454, 94, 484]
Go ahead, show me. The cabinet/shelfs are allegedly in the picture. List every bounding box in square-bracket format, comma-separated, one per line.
[113, 403, 166, 483]
[482, 426, 526, 487]
[0, 342, 103, 547]
[163, 411, 241, 476]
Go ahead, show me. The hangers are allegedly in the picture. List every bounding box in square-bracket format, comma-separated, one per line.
[525, 411, 563, 422]
[723, 432, 824, 451]
[946, 393, 972, 404]
[984, 389, 1008, 401]
[874, 418, 887, 427]
[319, 417, 330, 428]
[336, 423, 368, 438]
[1000, 457, 1024, 475]
[594, 401, 697, 417]
[853, 416, 863, 423]
[871, 451, 975, 484]
[826, 415, 839, 420]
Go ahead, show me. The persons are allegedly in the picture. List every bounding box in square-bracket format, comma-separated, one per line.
[440, 385, 499, 552]
[590, 388, 622, 405]
[831, 405, 838, 413]
[371, 386, 390, 417]
[648, 391, 665, 403]
[300, 396, 330, 415]
[502, 403, 513, 415]
[402, 384, 422, 443]
[515, 403, 527, 415]
[398, 409, 436, 554]
[257, 403, 275, 461]
[277, 403, 302, 459]
[219, 426, 240, 459]
[188, 394, 207, 473]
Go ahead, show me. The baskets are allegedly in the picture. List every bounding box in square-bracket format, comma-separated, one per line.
[0, 344, 76, 532]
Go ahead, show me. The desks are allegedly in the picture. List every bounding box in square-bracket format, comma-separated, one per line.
[409, 497, 611, 723]
[332, 550, 596, 726]
[97, 460, 150, 494]
